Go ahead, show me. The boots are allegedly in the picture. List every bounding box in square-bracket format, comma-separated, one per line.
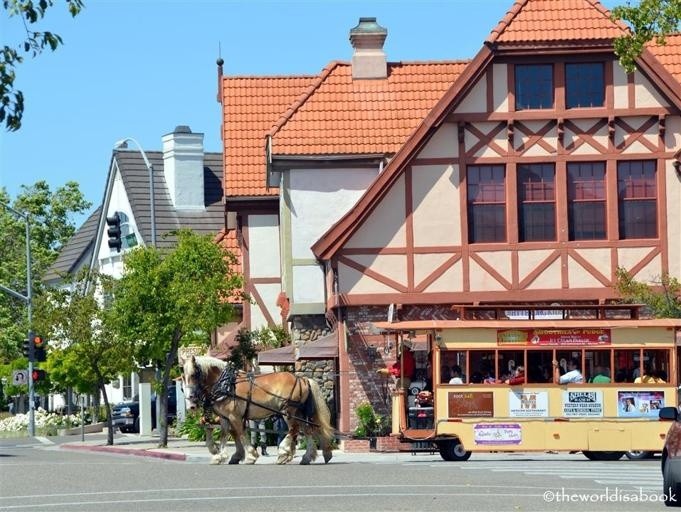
[251, 443, 269, 457]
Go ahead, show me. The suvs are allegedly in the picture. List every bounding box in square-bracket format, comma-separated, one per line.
[113, 383, 178, 433]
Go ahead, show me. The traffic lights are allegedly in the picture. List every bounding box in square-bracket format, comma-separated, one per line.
[36, 346, 47, 362]
[22, 338, 30, 357]
[34, 335, 45, 344]
[33, 369, 46, 381]
[106, 211, 123, 253]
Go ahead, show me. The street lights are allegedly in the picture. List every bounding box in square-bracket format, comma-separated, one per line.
[114, 137, 158, 250]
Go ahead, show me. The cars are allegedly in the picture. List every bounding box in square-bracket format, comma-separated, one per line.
[659, 401, 681, 506]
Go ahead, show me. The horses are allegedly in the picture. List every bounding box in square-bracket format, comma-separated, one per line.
[172, 354, 335, 466]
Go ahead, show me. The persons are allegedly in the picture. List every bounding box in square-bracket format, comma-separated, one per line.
[422, 351, 668, 391]
[640, 402, 650, 413]
[377, 338, 417, 431]
[651, 400, 661, 409]
[623, 399, 634, 411]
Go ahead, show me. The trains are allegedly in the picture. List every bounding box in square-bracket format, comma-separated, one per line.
[378, 316, 681, 467]
